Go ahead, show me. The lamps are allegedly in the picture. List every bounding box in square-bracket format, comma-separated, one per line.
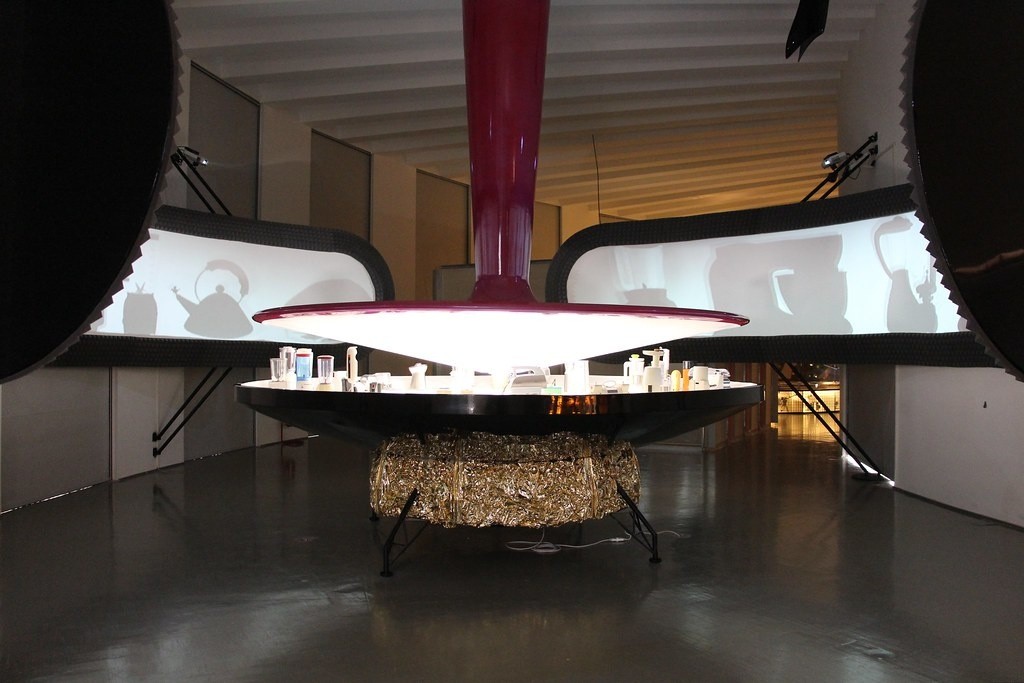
[251, 0, 751, 373]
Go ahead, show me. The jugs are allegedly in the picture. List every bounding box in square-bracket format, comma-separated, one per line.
[624, 354, 644, 394]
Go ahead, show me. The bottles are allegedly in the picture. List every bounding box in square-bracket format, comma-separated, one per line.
[296, 347, 313, 376]
[682, 361, 691, 390]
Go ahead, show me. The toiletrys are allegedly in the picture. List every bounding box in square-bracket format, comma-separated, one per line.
[623, 346, 709, 393]
[346, 346, 358, 380]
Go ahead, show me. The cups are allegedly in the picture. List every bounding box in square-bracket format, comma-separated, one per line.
[564, 371, 585, 395]
[279, 346, 296, 373]
[294, 357, 311, 381]
[317, 355, 334, 384]
[270, 357, 287, 382]
